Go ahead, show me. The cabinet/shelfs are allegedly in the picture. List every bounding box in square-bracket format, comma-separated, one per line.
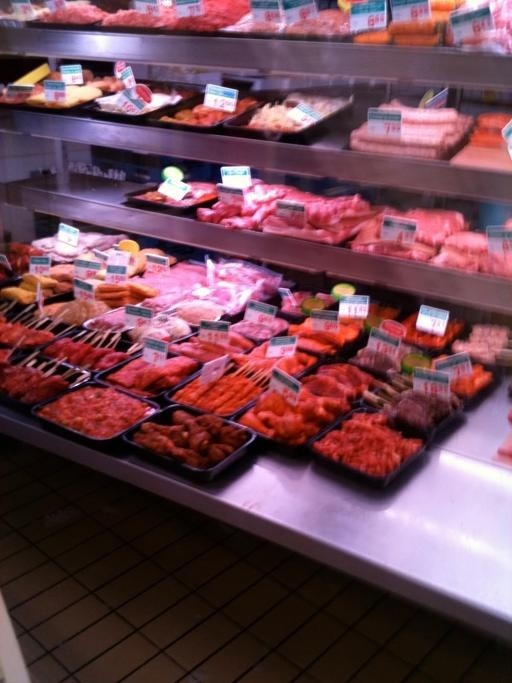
[0, 24, 511, 639]
